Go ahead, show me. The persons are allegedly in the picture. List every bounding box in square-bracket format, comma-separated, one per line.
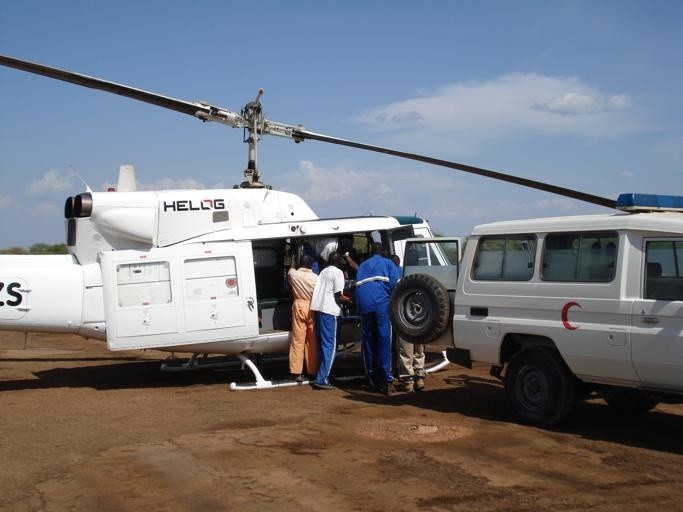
[282, 233, 428, 393]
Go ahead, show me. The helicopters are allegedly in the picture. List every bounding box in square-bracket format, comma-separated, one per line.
[1, 51, 621, 394]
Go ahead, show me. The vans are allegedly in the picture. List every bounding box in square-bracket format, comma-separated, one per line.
[387, 192, 682, 429]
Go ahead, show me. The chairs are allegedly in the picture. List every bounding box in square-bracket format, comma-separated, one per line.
[646, 263, 662, 278]
[255, 265, 293, 308]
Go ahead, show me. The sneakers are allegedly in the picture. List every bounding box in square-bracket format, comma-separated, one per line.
[287, 374, 336, 389]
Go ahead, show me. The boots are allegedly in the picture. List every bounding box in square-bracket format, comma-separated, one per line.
[383, 369, 425, 395]
